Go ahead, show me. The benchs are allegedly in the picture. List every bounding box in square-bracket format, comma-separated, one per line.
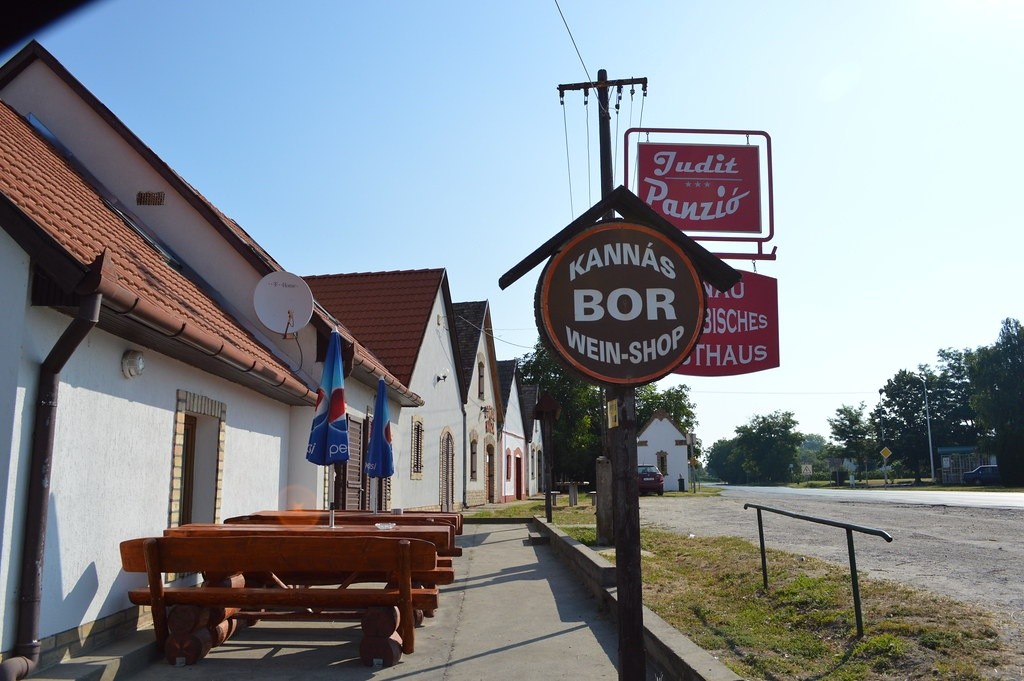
[544, 491, 560, 506]
[119, 536, 439, 666]
[411, 547, 463, 586]
[588, 491, 597, 505]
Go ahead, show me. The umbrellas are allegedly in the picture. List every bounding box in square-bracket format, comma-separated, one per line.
[364, 376, 395, 478]
[306, 324, 349, 467]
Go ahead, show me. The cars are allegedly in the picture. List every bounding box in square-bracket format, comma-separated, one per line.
[962, 464, 1002, 487]
[638, 463, 664, 497]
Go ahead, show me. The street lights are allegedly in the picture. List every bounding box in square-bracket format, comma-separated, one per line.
[879, 388, 888, 486]
[919, 377, 936, 485]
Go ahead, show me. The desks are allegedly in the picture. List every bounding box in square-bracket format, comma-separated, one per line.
[557, 482, 589, 506]
[163, 511, 461, 549]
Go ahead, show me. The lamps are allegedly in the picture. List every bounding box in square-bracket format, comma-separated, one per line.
[436, 368, 449, 381]
